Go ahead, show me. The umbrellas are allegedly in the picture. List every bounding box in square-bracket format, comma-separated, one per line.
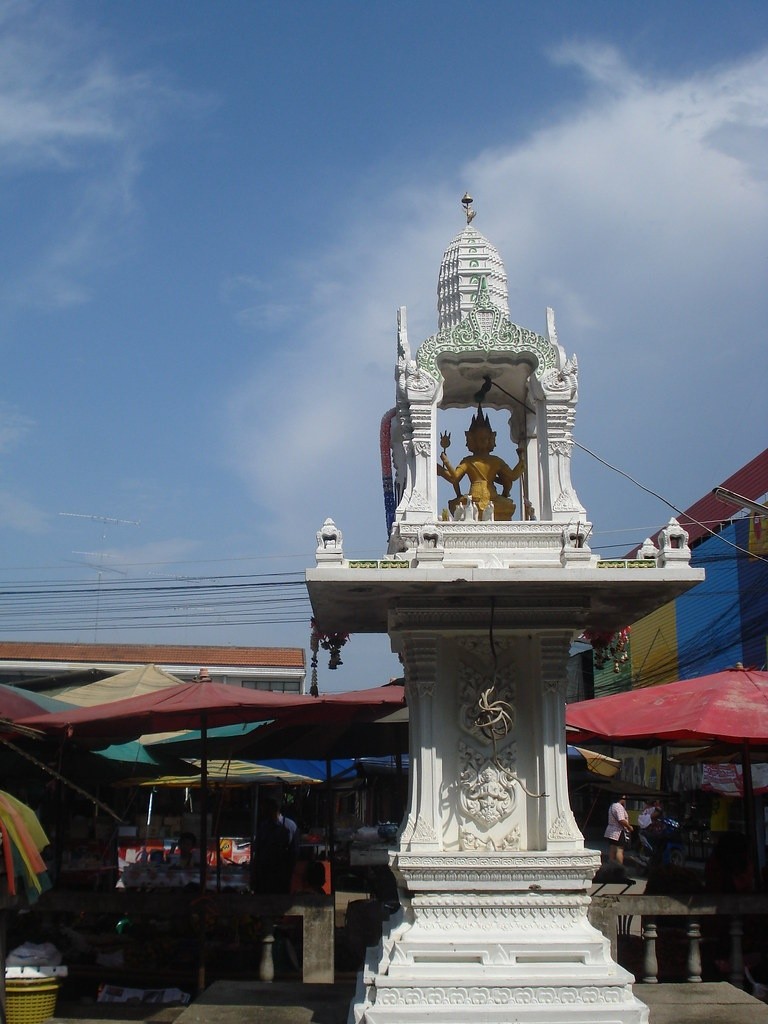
[1, 661, 767, 992]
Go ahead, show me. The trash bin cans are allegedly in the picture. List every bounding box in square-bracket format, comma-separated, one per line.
[378, 823, 398, 845]
[5, 977, 59, 1024]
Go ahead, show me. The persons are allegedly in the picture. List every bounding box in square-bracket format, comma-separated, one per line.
[638, 799, 667, 857]
[439, 406, 528, 521]
[165, 833, 206, 893]
[250, 797, 299, 893]
[604, 793, 635, 863]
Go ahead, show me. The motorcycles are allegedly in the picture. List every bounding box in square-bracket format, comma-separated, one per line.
[628, 815, 710, 877]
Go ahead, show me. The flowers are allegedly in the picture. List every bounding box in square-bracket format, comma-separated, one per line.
[310, 617, 348, 697]
[595, 626, 630, 673]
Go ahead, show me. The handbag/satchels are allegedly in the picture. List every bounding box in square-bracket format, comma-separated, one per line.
[611, 806, 632, 845]
